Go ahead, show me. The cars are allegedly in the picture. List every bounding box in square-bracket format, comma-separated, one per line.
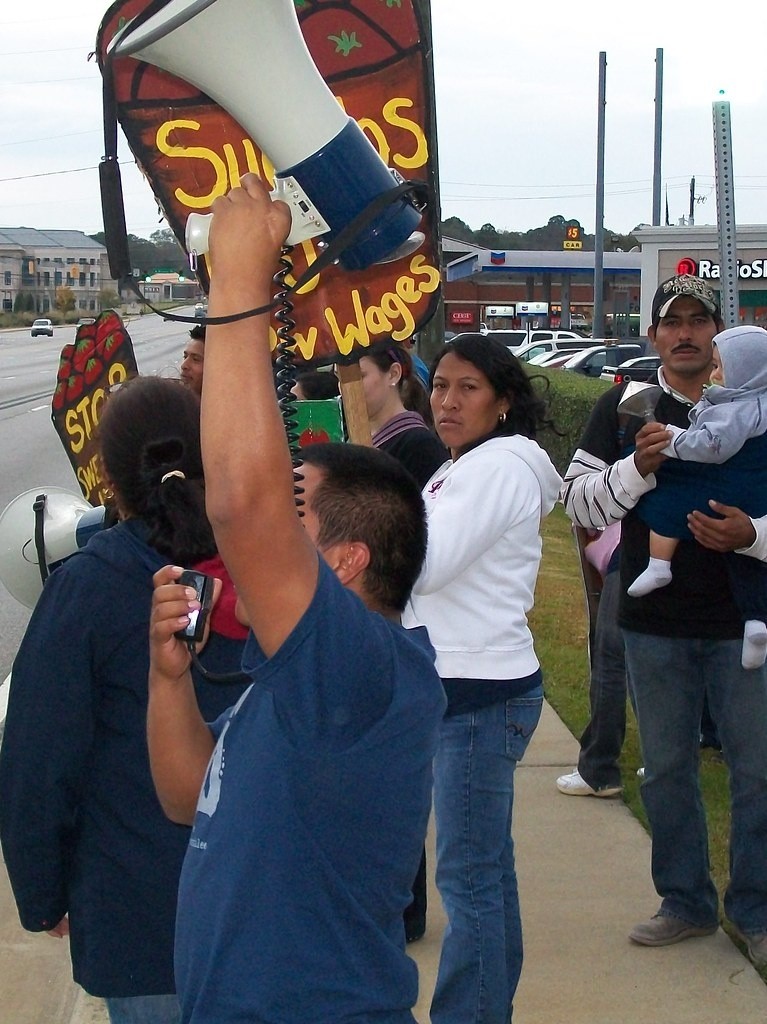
[477, 304, 668, 385]
[195, 308, 206, 318]
[30, 318, 53, 337]
[76, 318, 97, 334]
[194, 302, 204, 310]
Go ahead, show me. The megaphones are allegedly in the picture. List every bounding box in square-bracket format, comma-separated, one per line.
[0, 485, 119, 611]
[106, 0, 422, 271]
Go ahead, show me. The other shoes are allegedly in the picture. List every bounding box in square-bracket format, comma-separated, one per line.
[700, 733, 710, 749]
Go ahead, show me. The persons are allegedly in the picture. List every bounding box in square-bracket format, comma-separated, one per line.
[0, 172, 566, 1024]
[555, 276, 767, 972]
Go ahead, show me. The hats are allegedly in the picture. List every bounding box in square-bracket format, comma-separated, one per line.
[651, 272, 719, 325]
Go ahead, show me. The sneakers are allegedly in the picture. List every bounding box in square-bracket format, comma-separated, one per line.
[557, 772, 623, 797]
[630, 914, 719, 947]
[637, 768, 646, 780]
[734, 923, 767, 965]
